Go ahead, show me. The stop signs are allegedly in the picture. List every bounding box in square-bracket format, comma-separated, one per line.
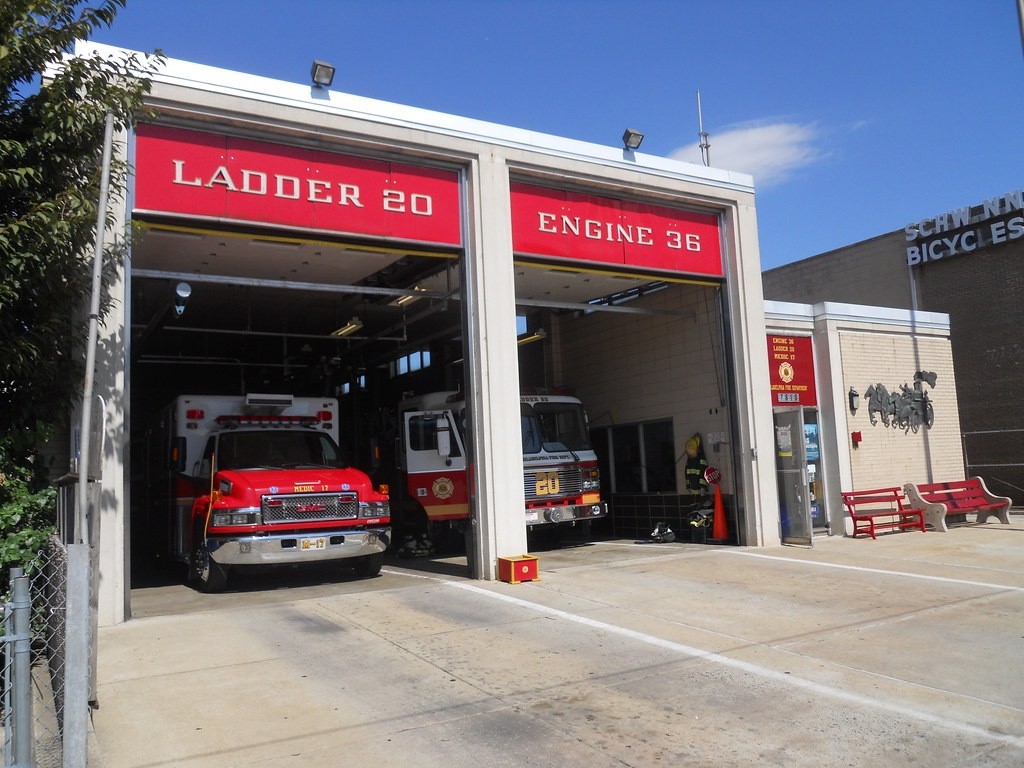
[704, 465, 722, 485]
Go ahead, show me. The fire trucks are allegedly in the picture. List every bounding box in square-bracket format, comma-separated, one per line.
[343, 383, 612, 541]
[158, 390, 394, 592]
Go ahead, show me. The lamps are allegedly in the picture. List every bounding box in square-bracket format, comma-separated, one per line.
[622, 129, 644, 151]
[310, 60, 335, 88]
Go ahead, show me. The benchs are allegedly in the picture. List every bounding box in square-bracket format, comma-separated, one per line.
[841, 487, 926, 540]
[904, 476, 1012, 532]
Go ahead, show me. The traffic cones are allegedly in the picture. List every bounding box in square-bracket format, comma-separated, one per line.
[708, 484, 735, 550]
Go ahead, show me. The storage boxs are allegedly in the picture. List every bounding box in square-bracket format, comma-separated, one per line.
[496, 554, 541, 585]
